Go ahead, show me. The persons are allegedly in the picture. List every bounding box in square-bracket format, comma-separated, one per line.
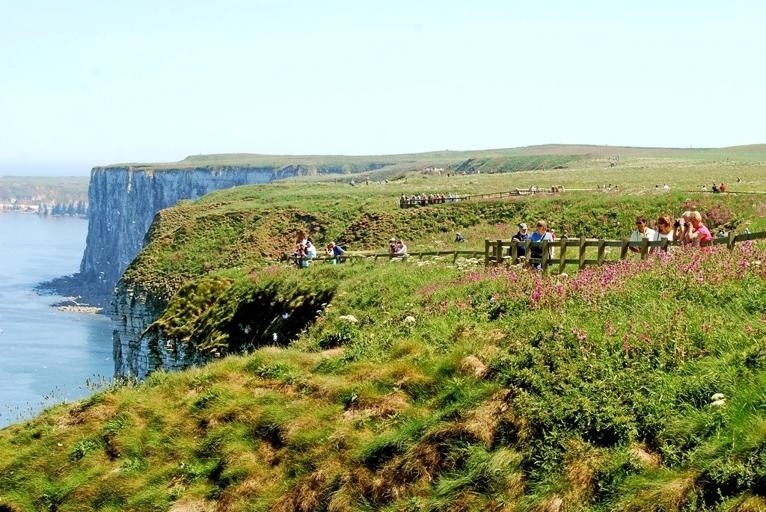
[509, 223, 531, 260]
[701, 185, 707, 191]
[321, 244, 337, 265]
[299, 232, 316, 268]
[388, 237, 397, 259]
[401, 193, 459, 204]
[529, 219, 554, 269]
[560, 230, 569, 239]
[712, 183, 720, 191]
[329, 241, 347, 264]
[292, 239, 301, 270]
[720, 183, 727, 191]
[628, 210, 712, 256]
[454, 232, 464, 242]
[393, 240, 407, 261]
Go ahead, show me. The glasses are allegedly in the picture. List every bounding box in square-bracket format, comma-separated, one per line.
[658, 222, 665, 225]
[636, 221, 642, 224]
[536, 224, 542, 227]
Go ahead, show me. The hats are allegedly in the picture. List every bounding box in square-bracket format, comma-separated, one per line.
[519, 223, 528, 230]
